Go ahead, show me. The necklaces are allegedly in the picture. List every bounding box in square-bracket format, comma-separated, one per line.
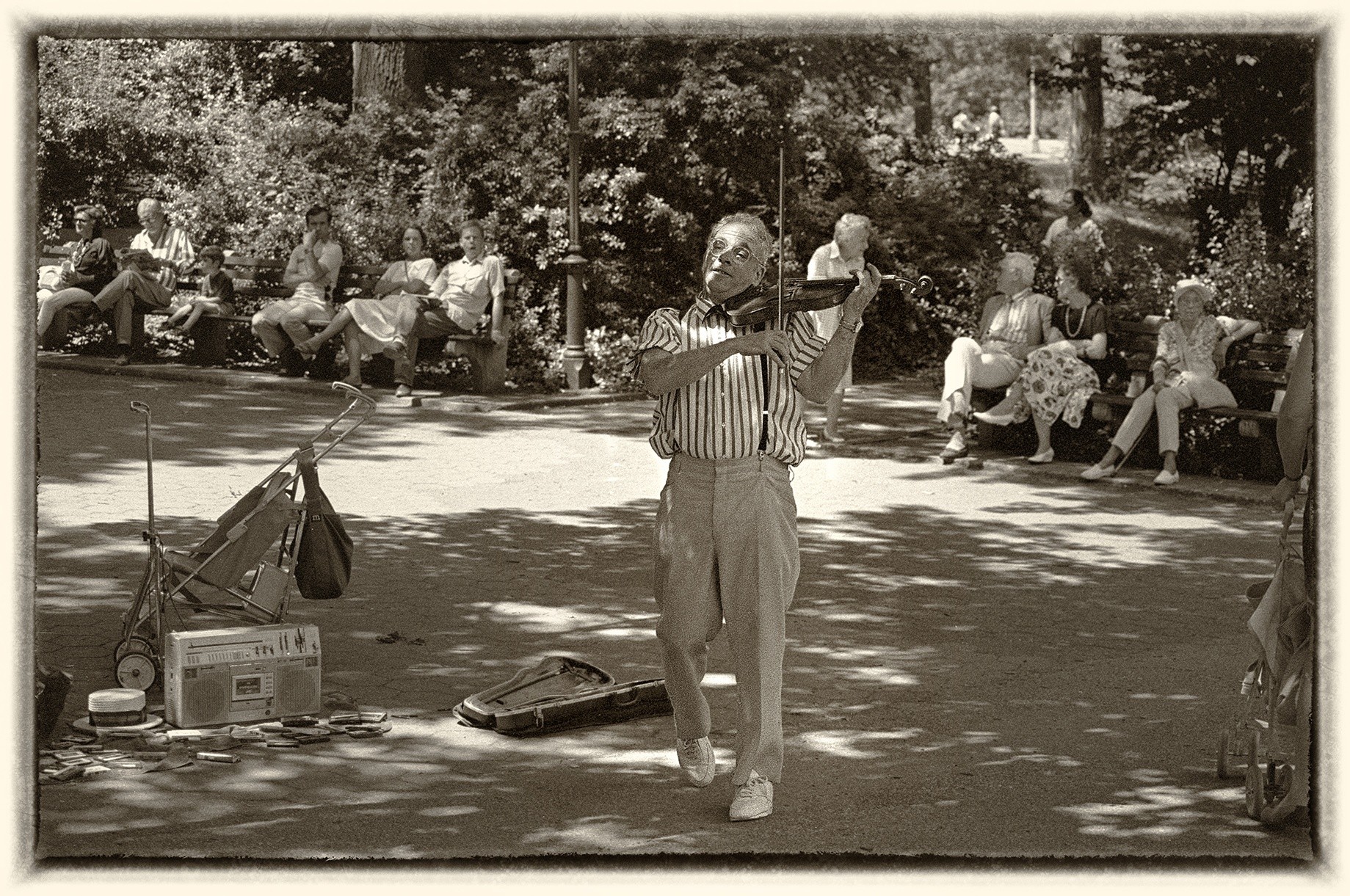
[1064, 302, 1087, 337]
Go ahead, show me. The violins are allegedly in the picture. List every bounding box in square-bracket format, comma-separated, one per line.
[727, 274, 934, 327]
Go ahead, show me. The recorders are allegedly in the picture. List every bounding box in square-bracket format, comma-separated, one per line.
[164, 622, 321, 729]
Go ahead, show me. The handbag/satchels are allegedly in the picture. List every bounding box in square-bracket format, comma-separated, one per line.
[1084, 350, 1131, 393]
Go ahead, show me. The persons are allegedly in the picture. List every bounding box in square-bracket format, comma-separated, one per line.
[935, 252, 1060, 459]
[384, 219, 508, 395]
[1263, 309, 1316, 824]
[157, 242, 237, 338]
[293, 224, 439, 389]
[34, 202, 116, 345]
[1081, 275, 1263, 486]
[66, 196, 196, 368]
[251, 205, 348, 379]
[645, 210, 885, 822]
[799, 212, 873, 443]
[1043, 187, 1114, 284]
[971, 257, 1114, 463]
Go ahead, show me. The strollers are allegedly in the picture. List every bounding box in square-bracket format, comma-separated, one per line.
[1215, 496, 1312, 821]
[110, 379, 378, 693]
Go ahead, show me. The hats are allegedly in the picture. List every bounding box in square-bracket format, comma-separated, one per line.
[1173, 279, 1210, 312]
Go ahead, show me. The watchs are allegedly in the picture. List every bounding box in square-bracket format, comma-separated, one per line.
[838, 316, 864, 334]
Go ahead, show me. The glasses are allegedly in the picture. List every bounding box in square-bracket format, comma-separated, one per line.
[708, 238, 765, 267]
[138, 209, 160, 225]
[1055, 276, 1074, 283]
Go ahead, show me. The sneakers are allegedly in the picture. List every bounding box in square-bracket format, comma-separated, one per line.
[676, 737, 714, 787]
[729, 768, 774, 822]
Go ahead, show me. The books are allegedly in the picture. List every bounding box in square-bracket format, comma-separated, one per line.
[119, 246, 159, 273]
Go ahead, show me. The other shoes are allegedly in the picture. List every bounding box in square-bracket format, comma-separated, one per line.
[382, 341, 411, 365]
[1079, 463, 1116, 479]
[296, 340, 319, 355]
[155, 322, 191, 338]
[939, 442, 969, 458]
[948, 409, 978, 424]
[395, 384, 410, 398]
[68, 304, 103, 327]
[338, 377, 364, 389]
[116, 353, 130, 365]
[823, 426, 844, 442]
[1154, 470, 1179, 484]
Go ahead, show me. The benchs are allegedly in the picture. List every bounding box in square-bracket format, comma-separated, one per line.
[64, 256, 520, 395]
[978, 320, 1298, 482]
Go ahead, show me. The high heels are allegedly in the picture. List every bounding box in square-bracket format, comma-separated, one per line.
[1028, 446, 1054, 463]
[972, 410, 1013, 426]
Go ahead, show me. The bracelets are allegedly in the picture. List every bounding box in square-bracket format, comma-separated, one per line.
[1284, 467, 1304, 484]
[304, 247, 314, 253]
[1229, 334, 1236, 342]
